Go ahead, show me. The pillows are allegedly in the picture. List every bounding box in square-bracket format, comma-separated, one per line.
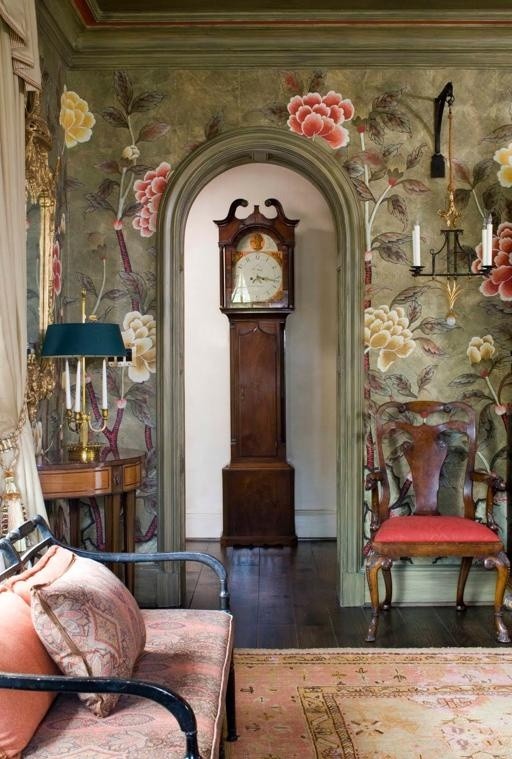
[0, 543, 82, 608]
[0, 582, 66, 759]
[28, 549, 147, 720]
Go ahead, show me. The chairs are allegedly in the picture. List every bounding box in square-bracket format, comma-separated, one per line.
[363, 395, 512, 646]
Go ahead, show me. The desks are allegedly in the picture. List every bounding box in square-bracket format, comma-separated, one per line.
[33, 446, 148, 604]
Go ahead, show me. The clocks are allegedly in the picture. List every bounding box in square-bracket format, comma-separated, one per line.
[216, 196, 304, 564]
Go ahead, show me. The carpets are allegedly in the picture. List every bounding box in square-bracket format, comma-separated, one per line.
[215, 645, 511, 758]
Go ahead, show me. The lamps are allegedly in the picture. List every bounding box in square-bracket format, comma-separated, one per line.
[409, 80, 495, 280]
[39, 286, 130, 460]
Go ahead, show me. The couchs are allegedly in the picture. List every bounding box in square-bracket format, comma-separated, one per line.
[0, 512, 239, 759]
